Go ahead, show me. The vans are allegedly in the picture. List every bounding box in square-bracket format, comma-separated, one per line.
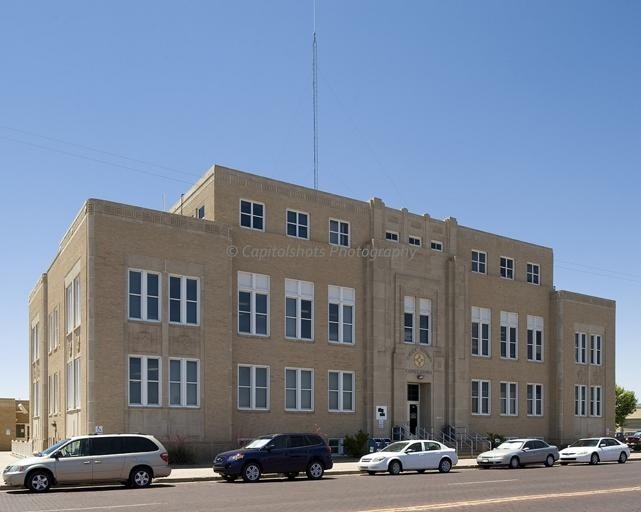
[3, 433, 171, 492]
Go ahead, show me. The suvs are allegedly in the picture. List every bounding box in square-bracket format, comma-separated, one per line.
[213, 432, 333, 482]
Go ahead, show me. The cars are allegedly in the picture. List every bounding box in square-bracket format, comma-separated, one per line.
[356, 440, 458, 474]
[615, 432, 641, 450]
[477, 438, 559, 468]
[555, 436, 631, 465]
